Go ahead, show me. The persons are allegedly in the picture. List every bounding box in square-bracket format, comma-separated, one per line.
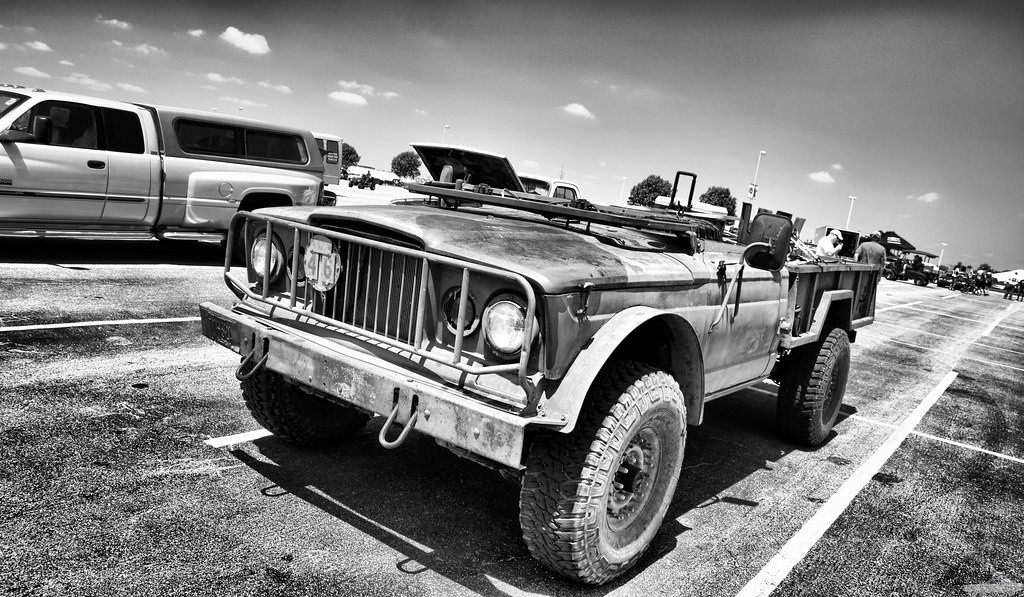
[816, 230, 843, 258]
[973, 266, 989, 296]
[1003, 272, 1024, 301]
[901, 255, 920, 273]
[949, 262, 972, 294]
[853, 231, 886, 282]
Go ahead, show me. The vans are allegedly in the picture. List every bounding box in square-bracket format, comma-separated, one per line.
[298, 129, 343, 187]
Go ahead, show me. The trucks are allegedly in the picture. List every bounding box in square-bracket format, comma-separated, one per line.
[200, 177, 879, 589]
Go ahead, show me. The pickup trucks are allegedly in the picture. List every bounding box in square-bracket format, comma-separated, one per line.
[0, 82, 324, 247]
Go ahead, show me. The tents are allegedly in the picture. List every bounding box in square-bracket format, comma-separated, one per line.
[859, 231, 938, 264]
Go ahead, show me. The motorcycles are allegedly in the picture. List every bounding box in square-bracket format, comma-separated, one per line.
[884, 248, 930, 286]
[348, 174, 375, 190]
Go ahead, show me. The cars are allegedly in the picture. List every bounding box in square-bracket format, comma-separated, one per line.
[938, 269, 968, 290]
[388, 142, 582, 206]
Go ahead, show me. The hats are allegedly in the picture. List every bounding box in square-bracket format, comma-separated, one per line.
[872, 231, 881, 240]
[830, 229, 844, 240]
[915, 255, 919, 257]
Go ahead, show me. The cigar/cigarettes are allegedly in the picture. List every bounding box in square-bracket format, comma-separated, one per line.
[837, 242, 839, 244]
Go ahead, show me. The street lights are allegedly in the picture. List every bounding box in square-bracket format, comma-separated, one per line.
[846, 195, 857, 230]
[751, 150, 766, 202]
[442, 126, 450, 144]
[937, 242, 948, 272]
